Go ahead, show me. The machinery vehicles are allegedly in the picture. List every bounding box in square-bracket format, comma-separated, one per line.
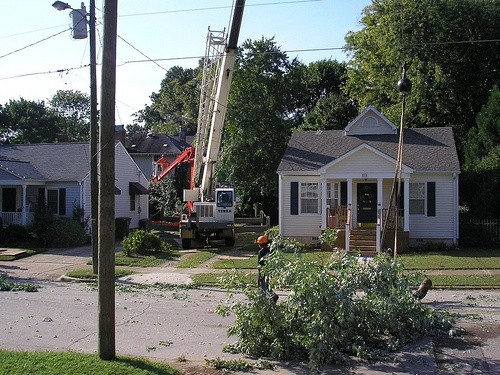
[174, 0, 245, 250]
[149, 146, 195, 231]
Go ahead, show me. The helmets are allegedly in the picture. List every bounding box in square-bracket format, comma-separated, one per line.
[257, 236, 267, 244]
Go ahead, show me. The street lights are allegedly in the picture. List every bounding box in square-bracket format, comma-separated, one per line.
[52, 0, 99, 281]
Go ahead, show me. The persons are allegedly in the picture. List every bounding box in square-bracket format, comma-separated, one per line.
[255, 236, 279, 304]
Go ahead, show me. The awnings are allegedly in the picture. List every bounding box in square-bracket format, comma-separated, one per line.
[129, 182, 149, 195]
